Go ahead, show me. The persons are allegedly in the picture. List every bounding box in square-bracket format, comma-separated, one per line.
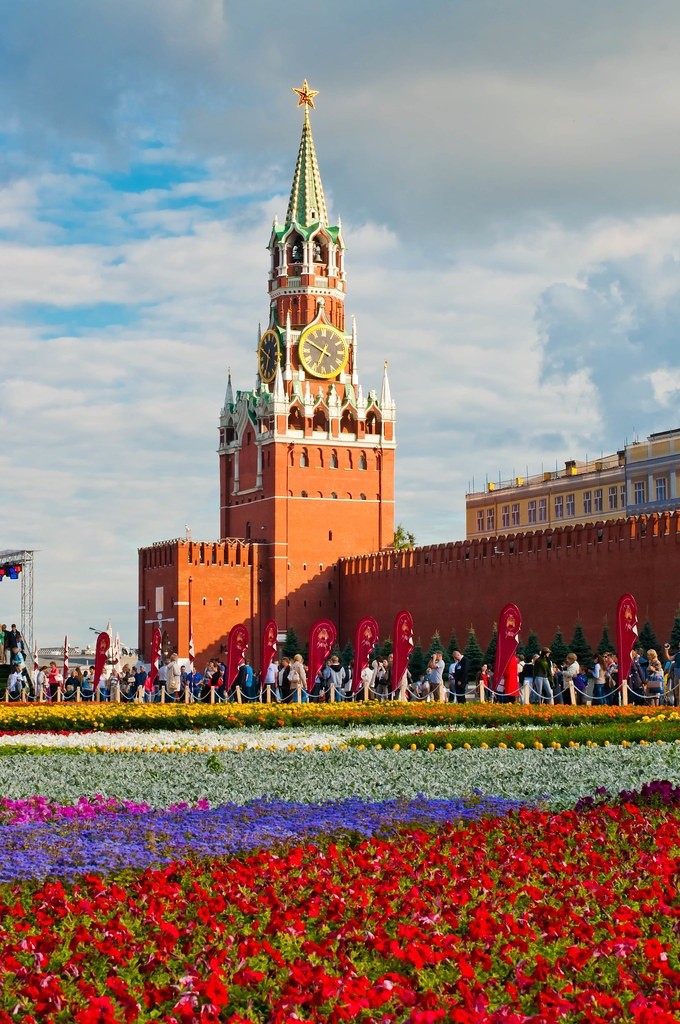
[0, 623, 680, 706]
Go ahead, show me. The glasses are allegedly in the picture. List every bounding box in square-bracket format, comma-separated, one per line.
[602, 656, 608, 658]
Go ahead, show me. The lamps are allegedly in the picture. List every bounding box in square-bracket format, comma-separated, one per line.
[220, 643, 228, 654]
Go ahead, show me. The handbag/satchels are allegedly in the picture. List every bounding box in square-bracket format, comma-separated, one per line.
[608, 675, 616, 688]
[54, 674, 63, 681]
[647, 681, 662, 688]
[344, 679, 352, 692]
[379, 679, 388, 685]
[168, 675, 179, 689]
[127, 677, 136, 682]
[287, 670, 300, 683]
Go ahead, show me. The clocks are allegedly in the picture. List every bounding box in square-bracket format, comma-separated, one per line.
[257, 328, 280, 383]
[298, 324, 349, 379]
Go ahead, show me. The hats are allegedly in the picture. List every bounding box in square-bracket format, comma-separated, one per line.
[542, 647, 551, 653]
[217, 665, 225, 671]
